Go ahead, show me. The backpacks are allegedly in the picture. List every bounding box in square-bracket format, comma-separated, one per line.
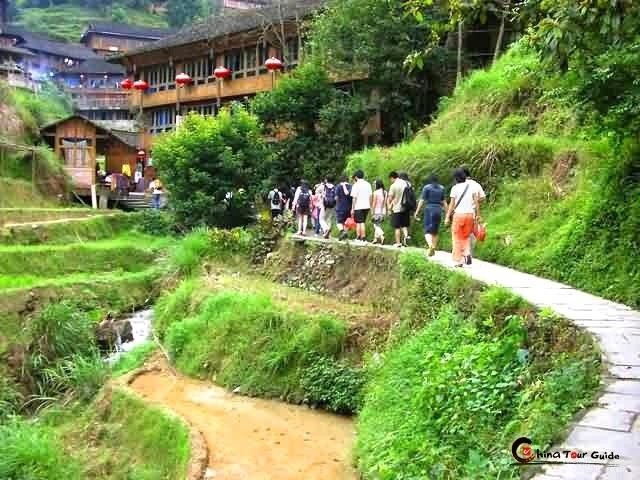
[402, 186, 417, 210]
[298, 192, 310, 208]
[273, 191, 280, 204]
[324, 185, 336, 209]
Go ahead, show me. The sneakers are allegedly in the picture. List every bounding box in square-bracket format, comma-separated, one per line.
[296, 228, 385, 246]
[392, 240, 472, 267]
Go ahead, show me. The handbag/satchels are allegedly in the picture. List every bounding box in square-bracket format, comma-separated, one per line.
[473, 222, 486, 241]
[371, 214, 383, 225]
[445, 209, 454, 228]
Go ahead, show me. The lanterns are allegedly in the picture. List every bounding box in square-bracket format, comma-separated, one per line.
[175, 72, 193, 88]
[214, 66, 231, 81]
[119, 79, 134, 90]
[264, 57, 283, 72]
[133, 80, 149, 95]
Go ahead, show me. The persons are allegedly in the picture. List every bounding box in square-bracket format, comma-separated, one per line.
[268, 164, 488, 271]
[146, 177, 167, 212]
